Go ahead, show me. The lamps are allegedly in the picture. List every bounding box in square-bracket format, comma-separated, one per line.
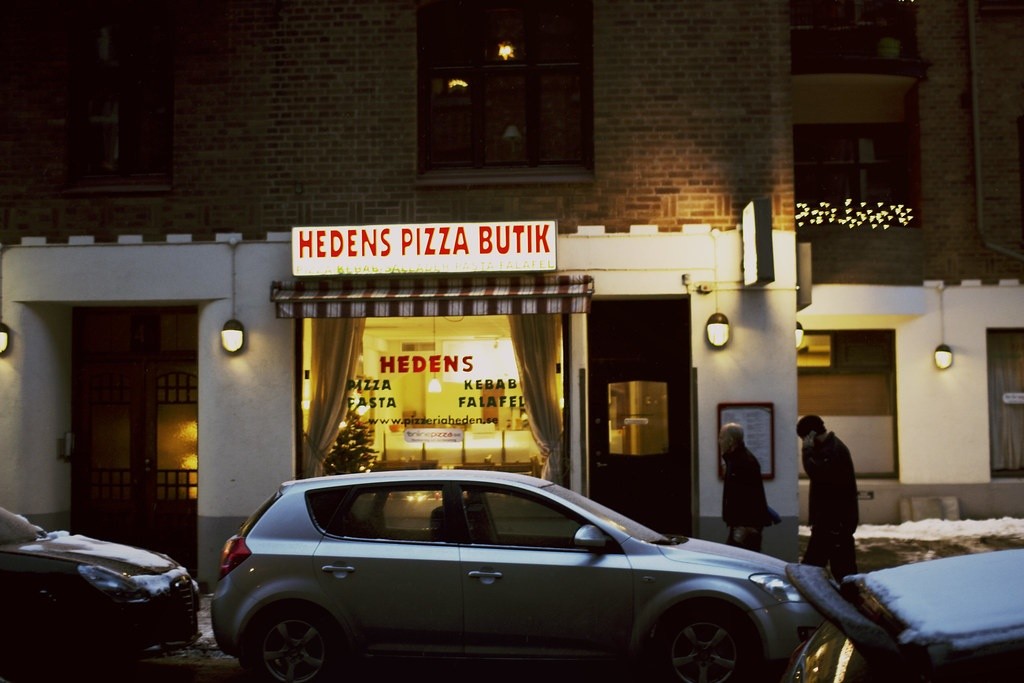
[708, 313, 730, 347]
[0, 322, 9, 354]
[936, 344, 953, 368]
[796, 321, 804, 347]
[429, 318, 442, 392]
[221, 319, 244, 351]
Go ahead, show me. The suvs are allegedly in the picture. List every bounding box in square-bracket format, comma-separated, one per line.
[210, 469, 841, 682]
[779, 549, 1024, 683]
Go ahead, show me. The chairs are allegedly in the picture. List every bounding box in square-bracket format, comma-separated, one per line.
[468, 503, 500, 547]
[431, 506, 450, 543]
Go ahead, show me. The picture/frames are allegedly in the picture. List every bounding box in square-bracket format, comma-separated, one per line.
[717, 403, 774, 481]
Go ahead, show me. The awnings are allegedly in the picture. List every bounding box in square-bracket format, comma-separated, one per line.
[270, 274, 593, 319]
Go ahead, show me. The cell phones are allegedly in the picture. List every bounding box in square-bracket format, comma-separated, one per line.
[806, 431, 816, 440]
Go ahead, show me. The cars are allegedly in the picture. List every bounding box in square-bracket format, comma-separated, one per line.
[0, 508, 203, 665]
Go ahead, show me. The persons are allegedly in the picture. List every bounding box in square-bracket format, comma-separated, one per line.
[795, 415, 860, 585]
[719, 423, 773, 551]
